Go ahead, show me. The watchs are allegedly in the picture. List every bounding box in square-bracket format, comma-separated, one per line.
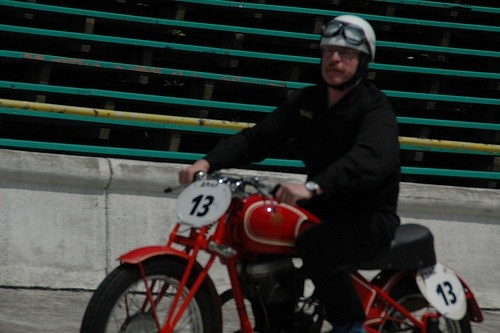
[306, 181, 318, 198]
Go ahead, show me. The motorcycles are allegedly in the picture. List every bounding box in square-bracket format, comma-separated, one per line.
[79, 171, 484, 333]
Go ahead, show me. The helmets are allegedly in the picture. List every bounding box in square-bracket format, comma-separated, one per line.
[320, 14, 376, 61]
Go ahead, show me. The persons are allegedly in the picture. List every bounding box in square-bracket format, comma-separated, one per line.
[178, 15, 402, 333]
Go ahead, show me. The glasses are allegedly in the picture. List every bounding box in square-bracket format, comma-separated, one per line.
[321, 19, 372, 60]
